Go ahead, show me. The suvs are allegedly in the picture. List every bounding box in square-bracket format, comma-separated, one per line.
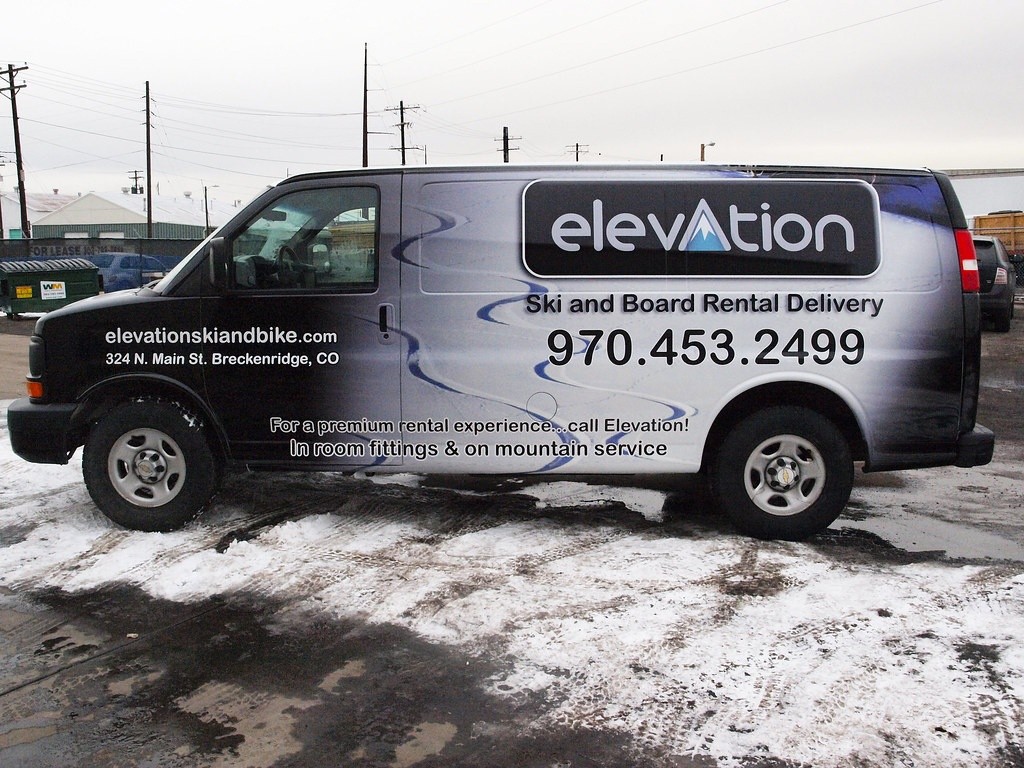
[972, 235, 1024, 331]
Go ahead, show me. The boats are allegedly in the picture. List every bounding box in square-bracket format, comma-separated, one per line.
[203, 183, 219, 237]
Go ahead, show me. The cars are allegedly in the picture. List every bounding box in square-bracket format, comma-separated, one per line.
[93, 252, 168, 293]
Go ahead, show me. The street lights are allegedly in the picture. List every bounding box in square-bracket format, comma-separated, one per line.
[700, 142, 716, 161]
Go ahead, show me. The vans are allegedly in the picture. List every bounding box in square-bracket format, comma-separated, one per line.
[5, 158, 998, 540]
